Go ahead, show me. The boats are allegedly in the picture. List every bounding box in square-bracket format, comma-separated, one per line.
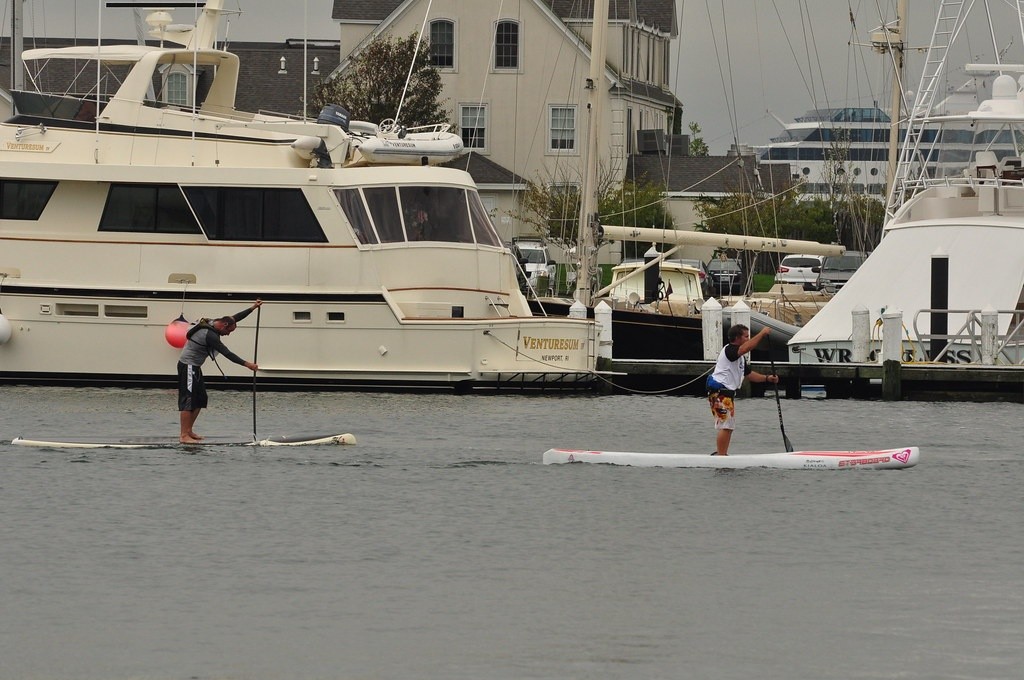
[0, 0, 625, 393]
[784, 0, 1023, 400]
[355, 130, 465, 164]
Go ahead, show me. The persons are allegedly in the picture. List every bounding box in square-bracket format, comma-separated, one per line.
[705, 324, 779, 456]
[407, 210, 431, 241]
[177, 299, 263, 443]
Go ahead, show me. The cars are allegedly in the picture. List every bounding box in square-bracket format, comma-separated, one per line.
[817, 251, 871, 288]
[705, 258, 745, 293]
[773, 254, 827, 291]
[511, 240, 557, 293]
[665, 258, 713, 295]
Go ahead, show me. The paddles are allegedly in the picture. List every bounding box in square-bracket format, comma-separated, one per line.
[766, 333, 794, 453]
[252, 300, 262, 442]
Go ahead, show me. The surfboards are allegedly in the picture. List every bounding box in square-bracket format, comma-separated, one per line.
[542, 447, 921, 472]
[8, 432, 357, 447]
